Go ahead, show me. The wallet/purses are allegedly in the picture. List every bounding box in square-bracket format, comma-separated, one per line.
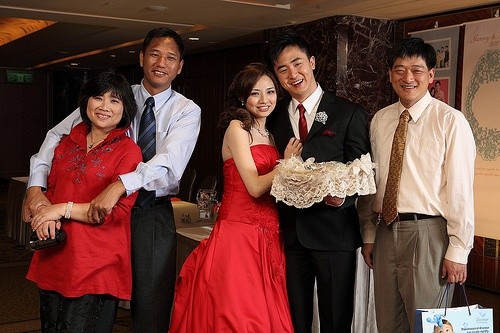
[30, 228, 66, 249]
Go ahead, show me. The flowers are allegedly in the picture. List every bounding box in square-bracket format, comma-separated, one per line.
[314, 111, 328, 124]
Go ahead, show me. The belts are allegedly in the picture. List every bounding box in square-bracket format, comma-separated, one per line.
[396, 213, 435, 221]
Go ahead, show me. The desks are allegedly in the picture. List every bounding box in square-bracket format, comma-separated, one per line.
[3, 176, 213, 275]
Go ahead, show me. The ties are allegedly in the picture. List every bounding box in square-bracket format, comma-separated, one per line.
[297, 104, 308, 141]
[382, 110, 412, 227]
[137, 97, 156, 212]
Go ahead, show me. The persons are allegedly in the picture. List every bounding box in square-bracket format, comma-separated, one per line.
[21, 27, 201, 333]
[435, 45, 449, 69]
[433, 320, 453, 333]
[167, 63, 291, 333]
[32, 69, 143, 333]
[430, 81, 445, 104]
[264, 39, 375, 333]
[357, 38, 475, 333]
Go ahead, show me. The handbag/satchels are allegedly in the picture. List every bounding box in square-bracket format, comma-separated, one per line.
[415, 280, 494, 333]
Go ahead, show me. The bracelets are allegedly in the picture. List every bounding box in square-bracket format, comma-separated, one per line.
[65, 201, 73, 219]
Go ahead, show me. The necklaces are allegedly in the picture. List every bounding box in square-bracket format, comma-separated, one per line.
[252, 125, 269, 137]
[89, 133, 106, 149]
